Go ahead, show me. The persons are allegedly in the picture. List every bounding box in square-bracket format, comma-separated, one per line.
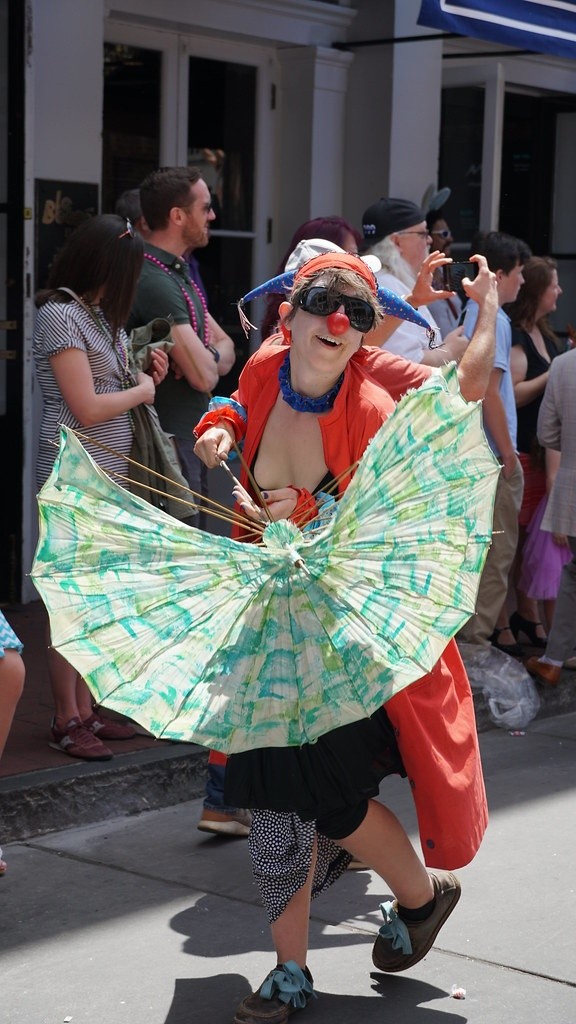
[193, 252, 489, 1024]
[116, 167, 235, 738]
[0, 609, 25, 874]
[260, 184, 576, 689]
[32, 214, 169, 761]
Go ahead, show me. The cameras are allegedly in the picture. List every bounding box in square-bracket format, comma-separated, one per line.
[443, 262, 478, 291]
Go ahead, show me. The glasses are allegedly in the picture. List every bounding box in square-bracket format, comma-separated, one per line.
[298, 286, 375, 333]
[175, 201, 212, 214]
[397, 229, 429, 240]
[431, 228, 451, 239]
[118, 217, 134, 240]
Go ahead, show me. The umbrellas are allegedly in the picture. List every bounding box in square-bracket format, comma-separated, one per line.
[24, 323, 504, 755]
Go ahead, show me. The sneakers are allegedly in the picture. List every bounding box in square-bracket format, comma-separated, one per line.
[48, 714, 113, 761]
[79, 709, 135, 739]
[196, 803, 254, 836]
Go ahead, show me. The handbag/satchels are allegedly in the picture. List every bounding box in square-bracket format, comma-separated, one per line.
[158, 431, 187, 475]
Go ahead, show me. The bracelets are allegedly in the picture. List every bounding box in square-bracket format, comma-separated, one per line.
[205, 345, 219, 363]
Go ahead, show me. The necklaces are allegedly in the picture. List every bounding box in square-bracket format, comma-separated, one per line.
[144, 252, 208, 347]
[278, 350, 345, 413]
[81, 296, 136, 434]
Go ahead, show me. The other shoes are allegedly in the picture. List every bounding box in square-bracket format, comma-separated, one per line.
[127, 715, 155, 738]
[234, 959, 318, 1024]
[372, 870, 461, 972]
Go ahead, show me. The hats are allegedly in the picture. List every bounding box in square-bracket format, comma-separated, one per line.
[284, 237, 382, 273]
[238, 253, 445, 353]
[357, 198, 425, 254]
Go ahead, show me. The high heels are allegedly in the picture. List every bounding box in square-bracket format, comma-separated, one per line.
[487, 625, 528, 664]
[510, 610, 549, 648]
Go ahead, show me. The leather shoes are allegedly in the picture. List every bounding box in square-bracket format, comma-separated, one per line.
[524, 653, 561, 689]
